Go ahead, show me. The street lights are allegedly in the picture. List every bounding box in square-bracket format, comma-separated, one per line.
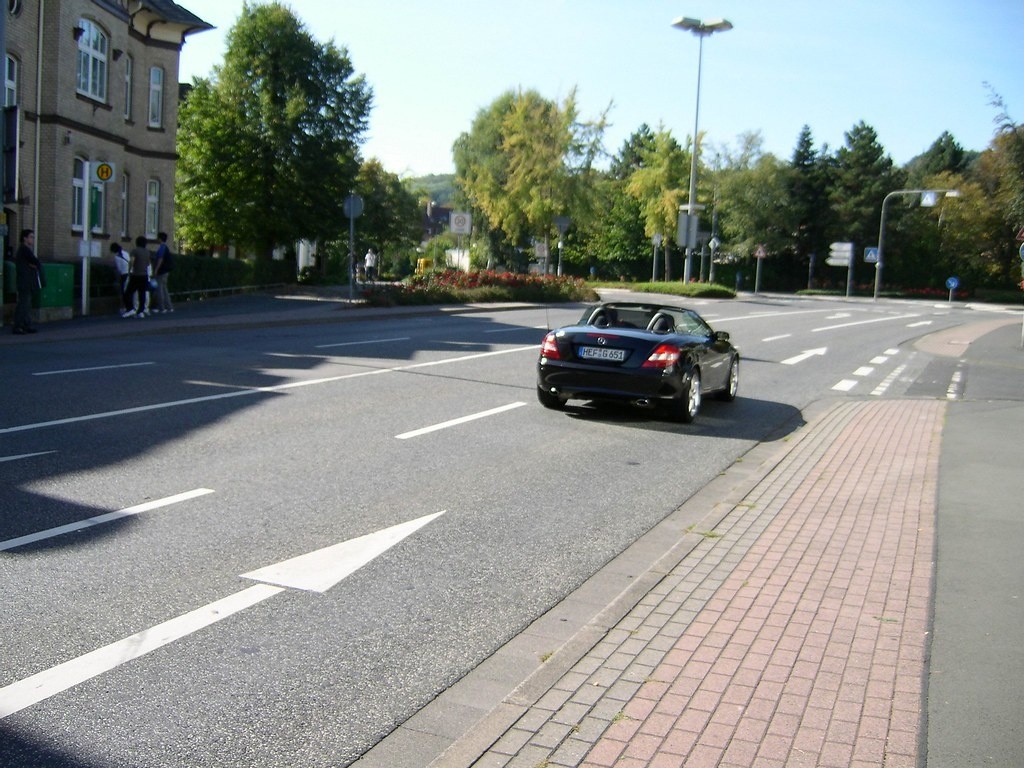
[672, 17, 735, 286]
[872, 189, 962, 302]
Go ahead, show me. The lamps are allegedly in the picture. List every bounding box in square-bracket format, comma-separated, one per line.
[65, 131, 72, 145]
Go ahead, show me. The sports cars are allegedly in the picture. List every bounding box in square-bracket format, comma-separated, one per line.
[535, 302, 741, 423]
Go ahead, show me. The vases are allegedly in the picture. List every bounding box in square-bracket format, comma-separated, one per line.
[113, 49, 123, 62]
[74, 27, 85, 41]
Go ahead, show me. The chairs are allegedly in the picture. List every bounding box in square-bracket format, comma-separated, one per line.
[606, 308, 625, 326]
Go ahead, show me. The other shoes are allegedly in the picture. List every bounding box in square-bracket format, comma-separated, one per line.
[133, 312, 145, 318]
[161, 309, 168, 313]
[122, 309, 136, 318]
[152, 309, 159, 313]
[12, 327, 26, 335]
[24, 326, 37, 333]
[168, 309, 174, 313]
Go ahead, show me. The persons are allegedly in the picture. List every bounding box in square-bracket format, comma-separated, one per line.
[10, 229, 47, 335]
[364, 249, 376, 282]
[152, 232, 174, 313]
[110, 236, 152, 318]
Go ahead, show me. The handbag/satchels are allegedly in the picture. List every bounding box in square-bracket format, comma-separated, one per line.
[146, 278, 159, 290]
[154, 247, 176, 275]
